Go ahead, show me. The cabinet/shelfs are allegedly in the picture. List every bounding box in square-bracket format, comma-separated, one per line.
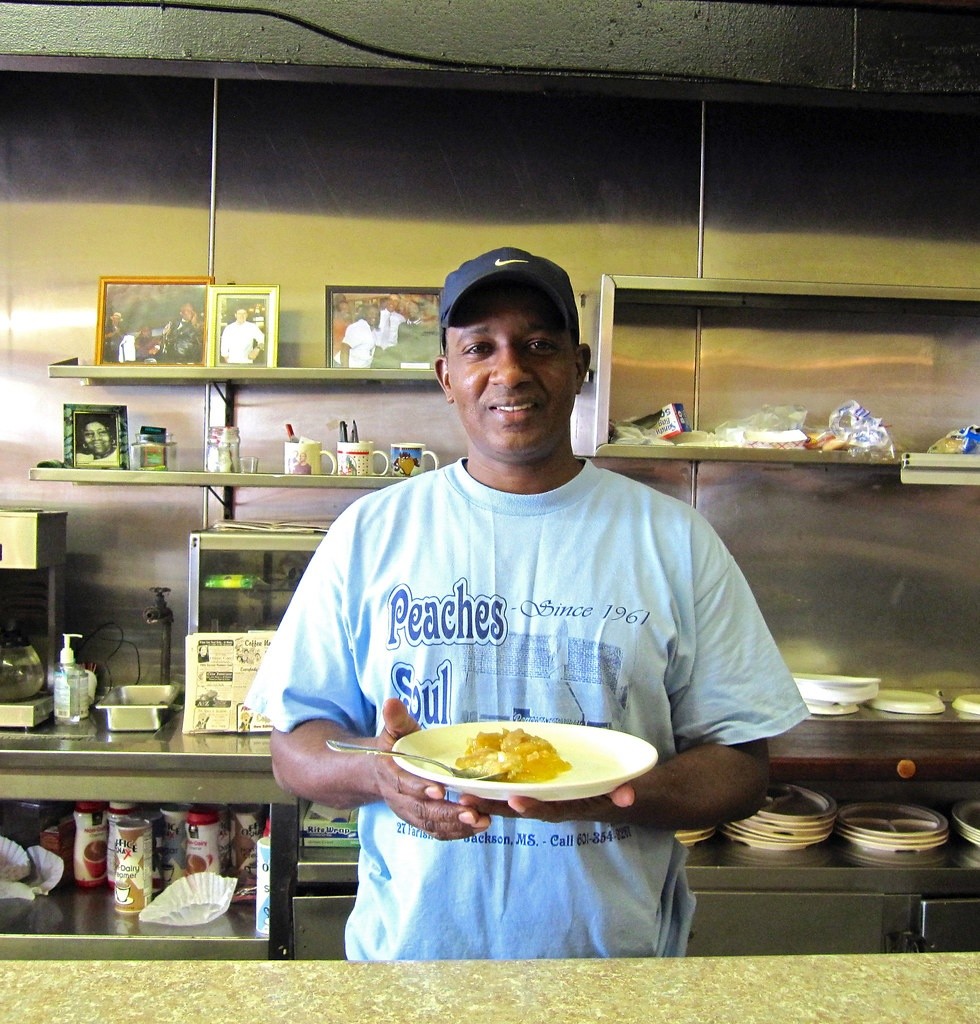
[1, 768, 299, 960]
[570, 274, 980, 466]
[292, 880, 980, 961]
[28, 358, 439, 489]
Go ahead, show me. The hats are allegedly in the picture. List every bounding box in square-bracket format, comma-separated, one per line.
[438, 246, 577, 331]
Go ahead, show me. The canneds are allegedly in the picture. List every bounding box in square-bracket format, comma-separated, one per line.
[126, 804, 262, 891]
[114, 819, 153, 914]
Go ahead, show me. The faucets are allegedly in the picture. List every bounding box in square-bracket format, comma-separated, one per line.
[143, 582, 176, 623]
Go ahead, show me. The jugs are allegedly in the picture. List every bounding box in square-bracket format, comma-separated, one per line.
[0, 618, 45, 704]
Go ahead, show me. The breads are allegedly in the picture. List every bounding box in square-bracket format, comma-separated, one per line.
[804, 431, 848, 449]
[925, 436, 964, 454]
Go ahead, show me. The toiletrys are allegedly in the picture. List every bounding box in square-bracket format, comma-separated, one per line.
[52, 633, 90, 720]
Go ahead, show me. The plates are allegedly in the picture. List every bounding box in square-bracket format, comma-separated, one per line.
[863, 690, 946, 715]
[951, 693, 979, 714]
[390, 721, 658, 802]
[674, 781, 979, 852]
[793, 703, 861, 717]
[720, 842, 979, 869]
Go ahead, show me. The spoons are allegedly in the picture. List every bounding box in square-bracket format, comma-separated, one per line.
[326, 738, 508, 779]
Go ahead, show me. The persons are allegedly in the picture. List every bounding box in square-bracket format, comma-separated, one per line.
[220, 305, 266, 364]
[102, 298, 202, 364]
[75, 410, 118, 465]
[244, 246, 808, 962]
[286, 450, 312, 475]
[332, 292, 442, 368]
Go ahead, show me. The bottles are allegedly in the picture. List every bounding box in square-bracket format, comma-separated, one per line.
[73, 802, 271, 936]
[76, 665, 88, 720]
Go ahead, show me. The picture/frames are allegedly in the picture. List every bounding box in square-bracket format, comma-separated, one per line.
[63, 404, 130, 471]
[94, 277, 216, 367]
[204, 285, 280, 369]
[325, 285, 444, 370]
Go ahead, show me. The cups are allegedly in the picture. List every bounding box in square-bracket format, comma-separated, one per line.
[132, 427, 438, 478]
[84, 669, 97, 705]
